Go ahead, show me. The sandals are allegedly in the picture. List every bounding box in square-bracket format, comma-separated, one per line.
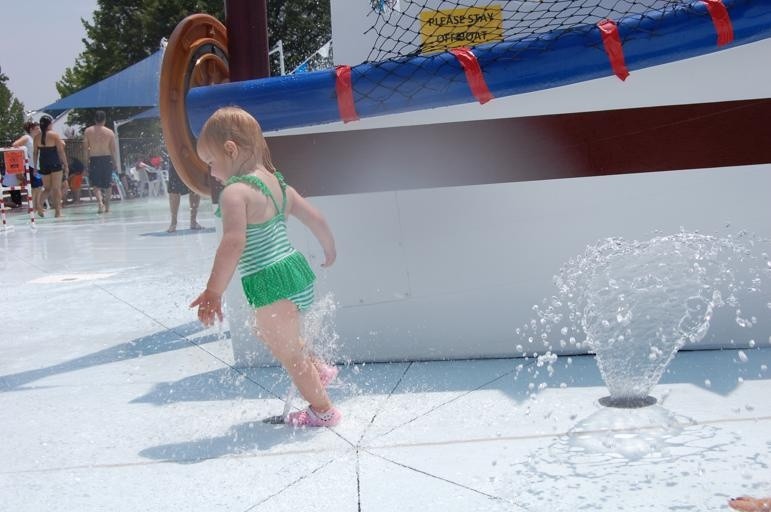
[288, 403, 342, 428]
[318, 364, 340, 388]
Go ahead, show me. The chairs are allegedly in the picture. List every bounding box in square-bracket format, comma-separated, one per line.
[129, 162, 169, 198]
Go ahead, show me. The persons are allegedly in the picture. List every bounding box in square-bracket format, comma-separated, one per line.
[0, 109, 166, 218]
[167, 158, 204, 231]
[187, 108, 342, 425]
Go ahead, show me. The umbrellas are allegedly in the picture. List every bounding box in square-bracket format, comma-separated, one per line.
[126, 106, 161, 122]
[38, 46, 163, 113]
